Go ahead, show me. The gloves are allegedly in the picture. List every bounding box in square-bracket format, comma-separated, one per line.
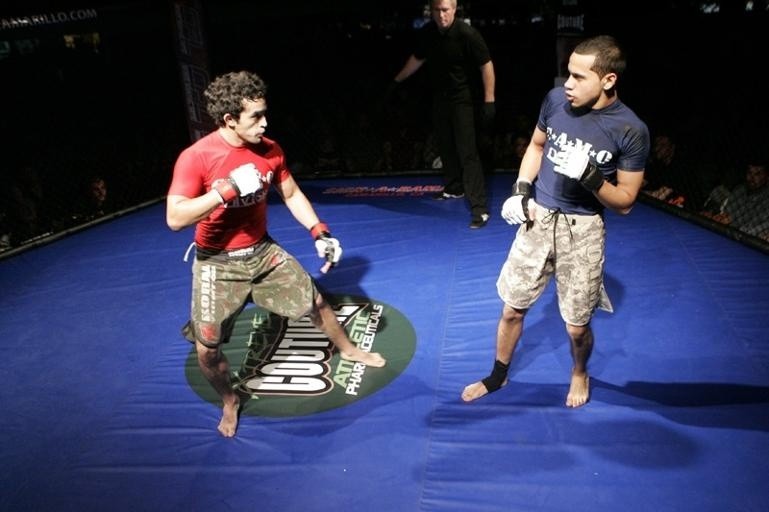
[309, 222, 343, 273]
[552, 144, 604, 192]
[501, 181, 536, 225]
[210, 161, 264, 208]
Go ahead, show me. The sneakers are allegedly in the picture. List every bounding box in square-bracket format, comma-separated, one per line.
[468, 212, 491, 230]
[430, 187, 465, 201]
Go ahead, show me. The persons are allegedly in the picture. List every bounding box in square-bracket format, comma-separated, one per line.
[165, 71, 387, 437]
[462, 29, 652, 407]
[391, 0, 497, 233]
[643, 107, 769, 244]
[0, 97, 108, 246]
